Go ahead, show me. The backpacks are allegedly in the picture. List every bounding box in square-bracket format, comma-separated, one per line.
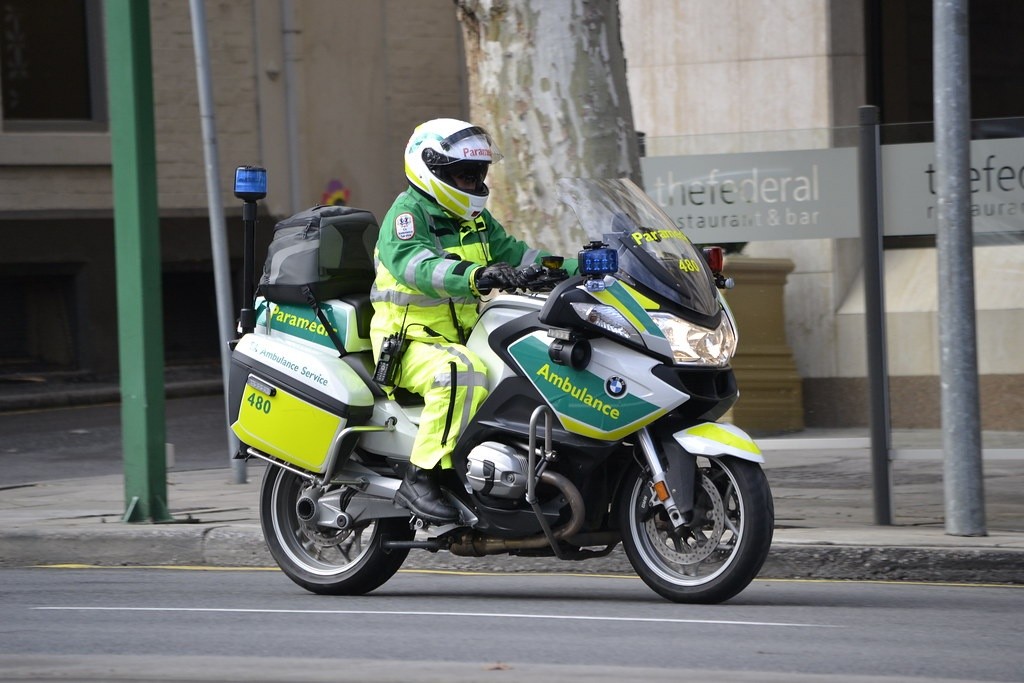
[258, 206, 380, 307]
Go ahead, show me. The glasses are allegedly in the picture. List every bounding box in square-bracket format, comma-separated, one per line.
[448, 164, 488, 183]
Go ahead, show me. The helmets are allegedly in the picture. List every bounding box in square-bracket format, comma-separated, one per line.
[404, 118, 504, 220]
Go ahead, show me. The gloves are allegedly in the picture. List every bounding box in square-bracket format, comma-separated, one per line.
[480, 262, 520, 293]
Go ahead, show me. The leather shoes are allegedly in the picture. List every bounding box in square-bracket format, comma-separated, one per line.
[394, 461, 460, 523]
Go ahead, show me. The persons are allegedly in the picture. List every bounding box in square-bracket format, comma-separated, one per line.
[370, 118, 581, 521]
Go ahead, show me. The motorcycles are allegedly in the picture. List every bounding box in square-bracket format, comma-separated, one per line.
[226, 165, 776, 606]
